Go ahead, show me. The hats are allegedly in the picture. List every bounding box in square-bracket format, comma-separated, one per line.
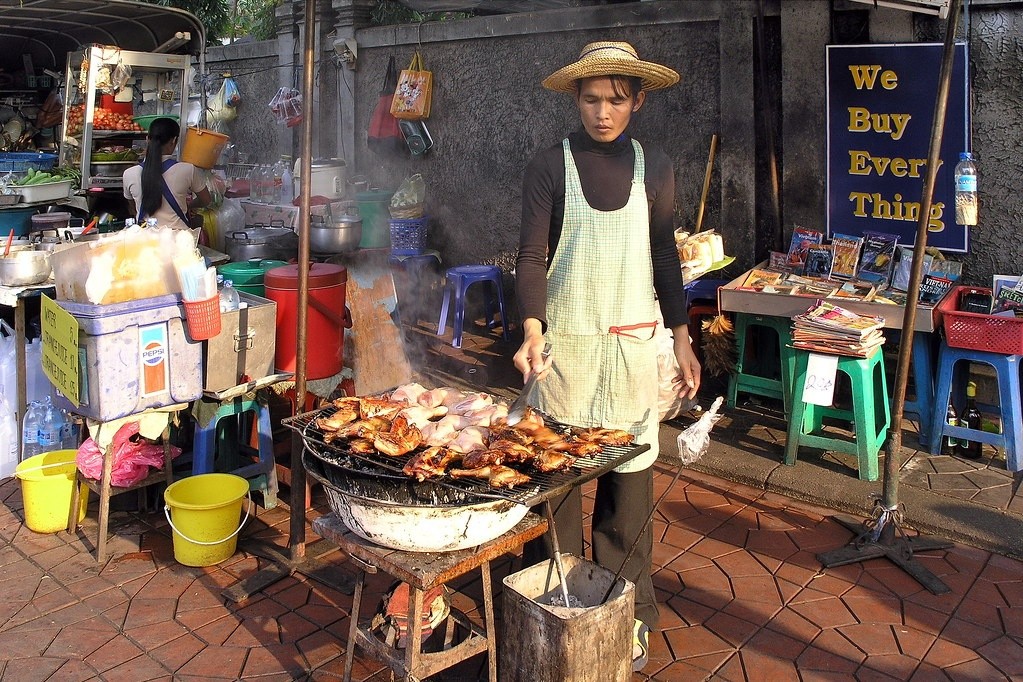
[541, 41, 679, 92]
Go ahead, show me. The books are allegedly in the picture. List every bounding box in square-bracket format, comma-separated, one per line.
[950, 275, 1023, 344]
[740, 224, 961, 307]
[785, 301, 886, 359]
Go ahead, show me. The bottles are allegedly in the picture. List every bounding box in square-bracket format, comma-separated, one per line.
[955, 153, 977, 225]
[941, 380, 982, 459]
[25, 338, 50, 400]
[122, 218, 159, 231]
[274, 155, 293, 204]
[22, 396, 78, 461]
[216, 274, 239, 313]
[250, 163, 274, 204]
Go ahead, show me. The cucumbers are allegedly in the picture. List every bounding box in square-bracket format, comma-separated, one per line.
[16, 168, 61, 185]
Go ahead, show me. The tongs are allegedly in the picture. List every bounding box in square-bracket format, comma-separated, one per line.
[506, 344, 553, 427]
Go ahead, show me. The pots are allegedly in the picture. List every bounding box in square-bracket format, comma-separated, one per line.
[224, 220, 299, 263]
[0, 218, 119, 255]
[309, 206, 363, 253]
[3, 115, 25, 142]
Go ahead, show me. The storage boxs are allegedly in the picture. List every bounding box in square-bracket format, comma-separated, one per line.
[48, 240, 276, 420]
[717, 249, 964, 333]
[941, 286, 1023, 356]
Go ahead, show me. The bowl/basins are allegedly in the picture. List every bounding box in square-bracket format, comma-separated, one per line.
[301, 411, 541, 552]
[73, 161, 139, 177]
[0, 195, 22, 204]
[131, 115, 180, 131]
[1, 250, 54, 286]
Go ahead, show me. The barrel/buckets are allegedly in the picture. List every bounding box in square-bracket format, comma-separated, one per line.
[164, 473, 251, 567]
[355, 188, 395, 249]
[182, 108, 230, 169]
[15, 449, 89, 534]
[217, 259, 352, 379]
[31, 212, 71, 232]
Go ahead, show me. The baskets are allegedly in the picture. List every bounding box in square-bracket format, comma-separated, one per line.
[388, 203, 425, 219]
[937, 285, 1023, 356]
[388, 216, 429, 256]
[181, 289, 222, 341]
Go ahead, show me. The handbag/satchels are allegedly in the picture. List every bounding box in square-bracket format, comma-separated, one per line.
[206, 74, 239, 128]
[367, 57, 411, 156]
[390, 51, 433, 119]
[225, 76, 242, 109]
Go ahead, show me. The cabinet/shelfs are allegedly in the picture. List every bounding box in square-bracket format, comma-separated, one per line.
[58, 46, 193, 191]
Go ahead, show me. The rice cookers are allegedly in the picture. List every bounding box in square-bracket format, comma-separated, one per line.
[294, 158, 348, 202]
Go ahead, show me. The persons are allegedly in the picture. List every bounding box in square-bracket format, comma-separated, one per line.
[512, 40, 701, 673]
[123, 118, 211, 231]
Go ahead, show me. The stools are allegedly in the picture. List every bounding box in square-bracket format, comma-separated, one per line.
[69, 377, 355, 566]
[878, 327, 942, 446]
[782, 345, 892, 480]
[437, 263, 511, 349]
[682, 278, 797, 422]
[931, 339, 1023, 471]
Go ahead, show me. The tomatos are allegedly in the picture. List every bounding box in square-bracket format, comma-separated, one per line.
[68, 103, 145, 134]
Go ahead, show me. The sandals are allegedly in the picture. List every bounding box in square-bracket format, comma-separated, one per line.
[632, 619, 649, 671]
